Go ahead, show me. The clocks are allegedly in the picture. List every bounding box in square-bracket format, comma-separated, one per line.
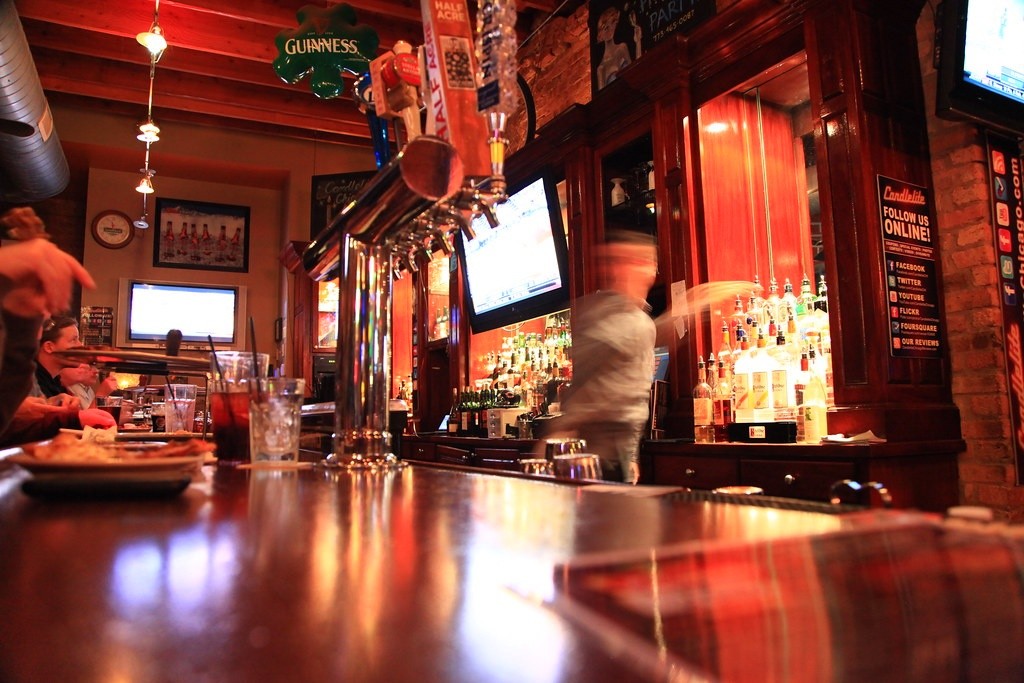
[93, 210, 135, 249]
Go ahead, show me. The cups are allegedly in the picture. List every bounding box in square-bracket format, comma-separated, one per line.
[248, 377, 306, 461]
[519, 438, 603, 479]
[151, 402, 166, 432]
[164, 384, 197, 435]
[210, 351, 270, 461]
[96, 396, 123, 426]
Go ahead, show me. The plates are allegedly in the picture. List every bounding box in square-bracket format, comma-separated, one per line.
[6, 448, 204, 500]
[115, 428, 151, 432]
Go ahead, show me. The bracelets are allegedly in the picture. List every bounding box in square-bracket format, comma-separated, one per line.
[78, 405, 81, 408]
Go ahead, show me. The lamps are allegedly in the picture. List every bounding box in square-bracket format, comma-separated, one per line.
[133, 0, 166, 229]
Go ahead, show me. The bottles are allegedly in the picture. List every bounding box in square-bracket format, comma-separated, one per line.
[132, 408, 153, 426]
[164, 221, 242, 263]
[486, 321, 572, 398]
[693, 274, 835, 444]
[447, 384, 497, 438]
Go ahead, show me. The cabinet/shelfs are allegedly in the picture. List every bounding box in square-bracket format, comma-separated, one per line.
[298, 410, 410, 464]
[401, 435, 969, 514]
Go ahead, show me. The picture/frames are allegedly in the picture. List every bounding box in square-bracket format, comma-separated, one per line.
[152, 197, 252, 271]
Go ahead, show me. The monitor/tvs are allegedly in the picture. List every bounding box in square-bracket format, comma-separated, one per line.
[456, 167, 571, 335]
[116, 277, 247, 360]
[933, 0, 1024, 137]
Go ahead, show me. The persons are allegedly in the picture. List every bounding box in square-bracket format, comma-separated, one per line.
[0, 208, 118, 447]
[537, 233, 656, 487]
